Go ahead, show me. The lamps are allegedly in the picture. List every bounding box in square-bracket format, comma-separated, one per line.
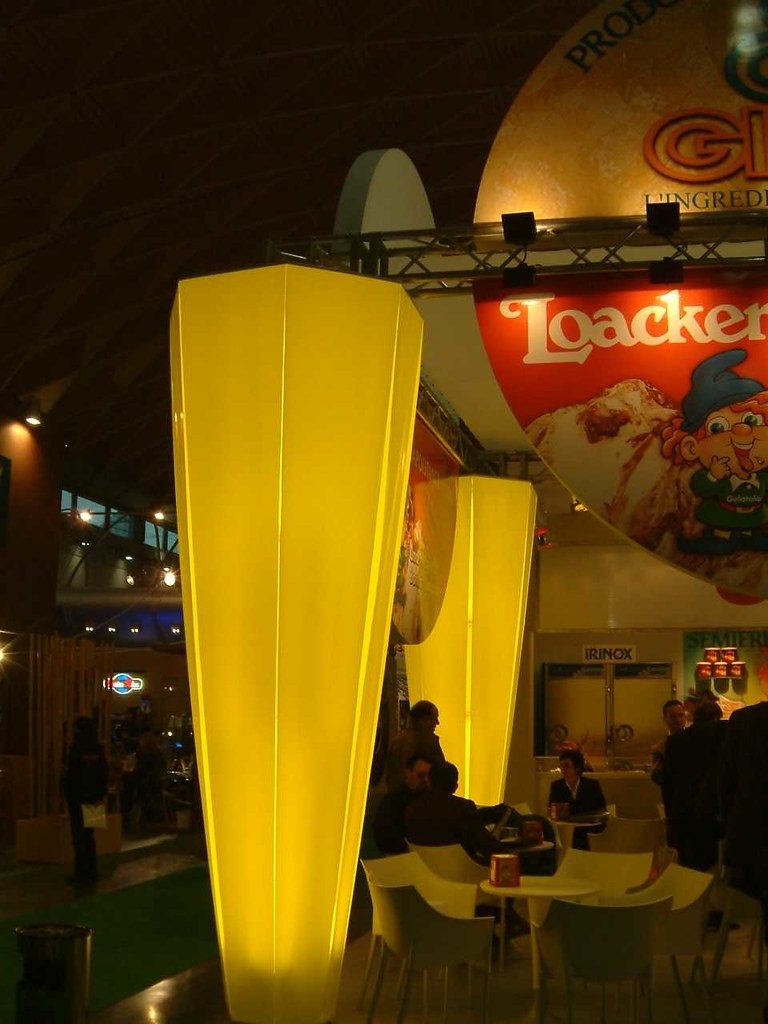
[502, 211, 540, 245]
[501, 265, 538, 288]
[25, 396, 43, 427]
[650, 258, 688, 284]
[646, 202, 681, 228]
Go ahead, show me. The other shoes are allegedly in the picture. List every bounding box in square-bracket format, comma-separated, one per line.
[704, 918, 740, 932]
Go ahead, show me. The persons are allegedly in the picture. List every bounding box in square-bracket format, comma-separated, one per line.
[110, 692, 170, 838]
[384, 701, 445, 794]
[548, 751, 608, 850]
[715, 700, 767, 1024]
[651, 700, 691, 770]
[371, 750, 435, 857]
[403, 763, 531, 962]
[58, 715, 109, 894]
[651, 704, 741, 933]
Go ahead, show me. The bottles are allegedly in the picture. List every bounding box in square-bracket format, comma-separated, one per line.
[730, 661, 746, 676]
[721, 647, 738, 661]
[704, 648, 721, 662]
[713, 662, 729, 677]
[696, 662, 712, 677]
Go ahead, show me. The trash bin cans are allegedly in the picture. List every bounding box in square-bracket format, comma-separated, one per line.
[15, 924, 92, 1024]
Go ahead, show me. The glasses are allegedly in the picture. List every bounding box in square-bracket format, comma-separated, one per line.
[558, 764, 575, 769]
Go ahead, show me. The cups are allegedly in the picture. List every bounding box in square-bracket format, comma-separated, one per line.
[503, 826, 518, 838]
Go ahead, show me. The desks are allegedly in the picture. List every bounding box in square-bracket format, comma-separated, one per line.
[555, 820, 601, 865]
[479, 876, 599, 1016]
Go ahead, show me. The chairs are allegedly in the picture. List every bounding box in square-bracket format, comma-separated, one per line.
[356, 798, 765, 1024]
